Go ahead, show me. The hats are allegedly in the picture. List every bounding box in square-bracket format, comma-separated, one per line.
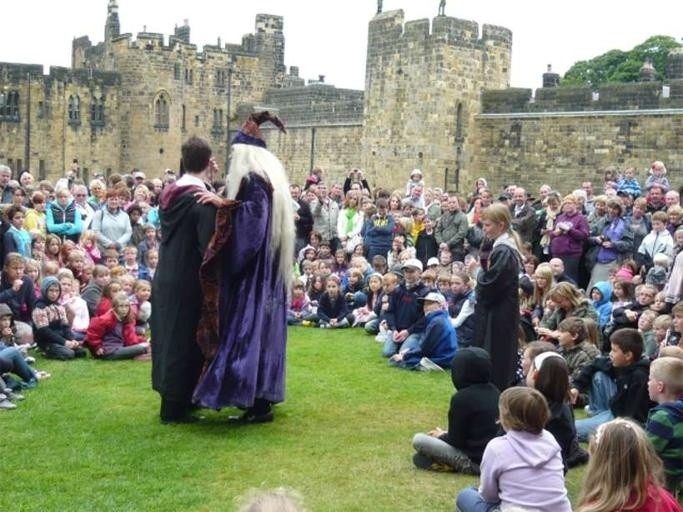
[131, 171, 146, 181]
[401, 258, 424, 272]
[231, 111, 287, 147]
[426, 257, 440, 267]
[75, 193, 88, 199]
[417, 292, 446, 306]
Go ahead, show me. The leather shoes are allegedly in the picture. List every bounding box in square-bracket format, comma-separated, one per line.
[229, 412, 276, 424]
[159, 414, 208, 425]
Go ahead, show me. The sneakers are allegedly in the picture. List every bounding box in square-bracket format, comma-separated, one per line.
[0, 399, 19, 410]
[419, 356, 444, 374]
[7, 393, 24, 403]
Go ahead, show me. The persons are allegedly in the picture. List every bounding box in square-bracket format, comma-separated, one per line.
[667, 205, 683, 231]
[389, 193, 401, 219]
[521, 341, 558, 387]
[512, 187, 535, 242]
[643, 357, 683, 492]
[437, 193, 448, 212]
[0, 301, 14, 355]
[548, 257, 576, 286]
[73, 182, 96, 231]
[287, 280, 315, 326]
[345, 269, 367, 297]
[90, 172, 174, 192]
[338, 190, 365, 237]
[412, 347, 504, 475]
[194, 109, 285, 424]
[128, 205, 143, 242]
[0, 252, 34, 333]
[151, 135, 220, 426]
[576, 418, 683, 512]
[423, 188, 440, 218]
[471, 203, 524, 383]
[588, 194, 607, 244]
[433, 187, 443, 198]
[118, 191, 129, 208]
[328, 182, 342, 206]
[87, 291, 152, 360]
[661, 227, 683, 303]
[625, 197, 652, 243]
[435, 197, 467, 259]
[659, 347, 683, 359]
[652, 315, 671, 349]
[412, 209, 426, 238]
[0, 348, 50, 409]
[406, 169, 424, 196]
[534, 265, 556, 296]
[672, 300, 683, 347]
[549, 194, 588, 280]
[289, 183, 314, 253]
[32, 277, 86, 362]
[27, 193, 45, 231]
[312, 167, 322, 182]
[418, 249, 454, 287]
[523, 255, 540, 279]
[392, 293, 459, 370]
[604, 167, 619, 196]
[416, 215, 438, 261]
[665, 190, 680, 209]
[557, 316, 592, 408]
[300, 175, 319, 207]
[383, 260, 432, 358]
[616, 167, 641, 197]
[466, 178, 516, 203]
[645, 162, 670, 192]
[363, 275, 382, 333]
[344, 168, 370, 197]
[646, 186, 665, 212]
[604, 282, 633, 335]
[637, 310, 659, 356]
[537, 281, 591, 339]
[363, 200, 394, 259]
[93, 189, 132, 250]
[526, 349, 578, 479]
[638, 211, 675, 286]
[571, 328, 653, 444]
[0, 165, 50, 201]
[654, 291, 667, 313]
[388, 231, 416, 270]
[444, 273, 476, 346]
[456, 387, 572, 512]
[532, 184, 552, 211]
[310, 182, 338, 243]
[612, 285, 659, 330]
[589, 281, 611, 334]
[581, 181, 595, 203]
[401, 203, 414, 230]
[572, 190, 589, 215]
[299, 233, 364, 280]
[381, 274, 398, 308]
[592, 197, 634, 280]
[369, 256, 387, 276]
[4, 206, 32, 254]
[47, 188, 81, 238]
[33, 235, 158, 293]
[316, 275, 354, 329]
